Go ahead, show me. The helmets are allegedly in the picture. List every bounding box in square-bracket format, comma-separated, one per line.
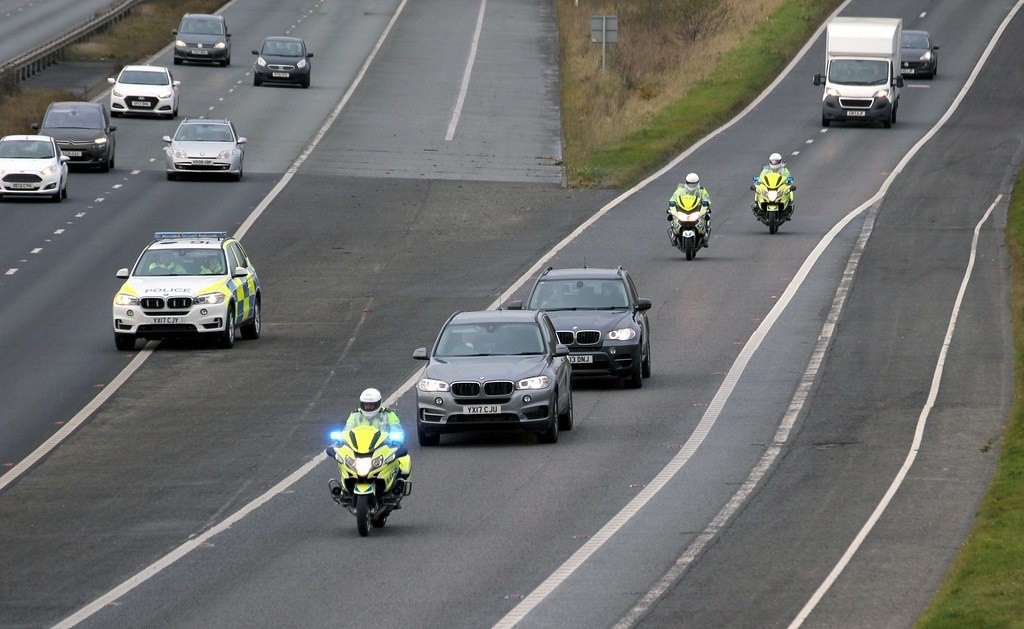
[684, 173, 699, 191]
[769, 153, 782, 169]
[359, 387, 382, 419]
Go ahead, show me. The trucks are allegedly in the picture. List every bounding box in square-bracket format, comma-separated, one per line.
[810, 16, 907, 129]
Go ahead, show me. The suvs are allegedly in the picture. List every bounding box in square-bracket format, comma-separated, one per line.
[415, 309, 582, 445]
[171, 14, 237, 69]
[163, 118, 247, 183]
[507, 266, 658, 386]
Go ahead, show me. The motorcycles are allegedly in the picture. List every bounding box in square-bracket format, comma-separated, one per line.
[327, 422, 417, 538]
[748, 169, 803, 237]
[664, 193, 714, 261]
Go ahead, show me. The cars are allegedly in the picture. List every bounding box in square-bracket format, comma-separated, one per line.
[250, 36, 314, 87]
[897, 30, 941, 82]
[0, 135, 70, 202]
[31, 101, 118, 172]
[112, 231, 263, 350]
[107, 64, 188, 118]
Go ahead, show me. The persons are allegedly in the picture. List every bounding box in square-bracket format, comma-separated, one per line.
[754, 152, 793, 219]
[541, 282, 567, 308]
[149, 252, 186, 274]
[340, 388, 412, 510]
[180, 126, 197, 141]
[669, 173, 711, 248]
[196, 255, 233, 274]
